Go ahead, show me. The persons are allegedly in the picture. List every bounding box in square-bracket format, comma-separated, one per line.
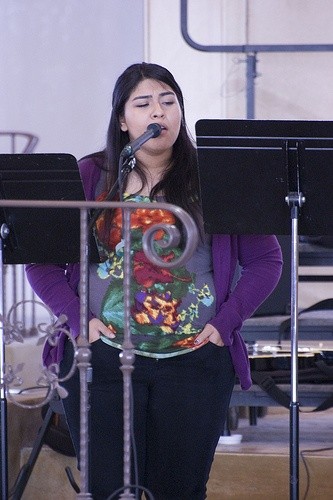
[23, 59, 283, 500]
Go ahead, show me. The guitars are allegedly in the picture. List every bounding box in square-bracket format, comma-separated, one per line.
[249, 344, 332, 371]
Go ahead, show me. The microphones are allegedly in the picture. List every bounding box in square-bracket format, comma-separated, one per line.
[120, 122, 161, 159]
